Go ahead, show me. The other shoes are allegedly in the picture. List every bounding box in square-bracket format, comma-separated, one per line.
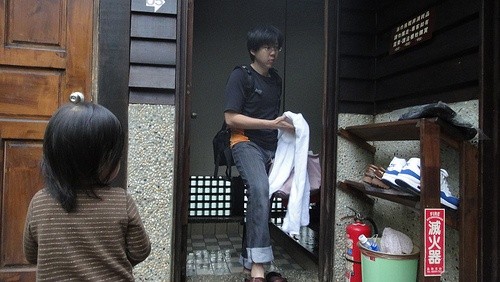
[266, 271, 287, 282]
[250, 276, 265, 282]
[363, 164, 378, 184]
[371, 166, 390, 189]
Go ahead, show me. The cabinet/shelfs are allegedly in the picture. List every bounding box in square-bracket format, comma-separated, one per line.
[335, 118, 480, 282]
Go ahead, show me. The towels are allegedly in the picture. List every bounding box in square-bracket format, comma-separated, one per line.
[267, 110, 311, 234]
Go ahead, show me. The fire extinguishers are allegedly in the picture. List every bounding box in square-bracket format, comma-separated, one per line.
[340, 206, 377, 281]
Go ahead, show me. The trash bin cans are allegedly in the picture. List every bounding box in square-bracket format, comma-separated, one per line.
[358, 238, 420, 281]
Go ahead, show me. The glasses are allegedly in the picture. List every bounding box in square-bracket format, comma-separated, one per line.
[262, 45, 283, 53]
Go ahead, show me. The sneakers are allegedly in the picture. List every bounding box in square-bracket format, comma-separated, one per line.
[396, 158, 458, 211]
[381, 156, 407, 191]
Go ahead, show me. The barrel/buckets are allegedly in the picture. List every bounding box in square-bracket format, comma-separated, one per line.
[358, 238, 420, 282]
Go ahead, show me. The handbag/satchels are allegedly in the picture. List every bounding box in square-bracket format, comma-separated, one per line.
[213, 123, 235, 167]
[399, 102, 477, 141]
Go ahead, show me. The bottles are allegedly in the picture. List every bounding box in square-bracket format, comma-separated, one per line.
[358, 235, 380, 252]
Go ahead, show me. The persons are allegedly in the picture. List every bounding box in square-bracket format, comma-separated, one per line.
[22, 101, 151, 282]
[224, 23, 296, 282]
